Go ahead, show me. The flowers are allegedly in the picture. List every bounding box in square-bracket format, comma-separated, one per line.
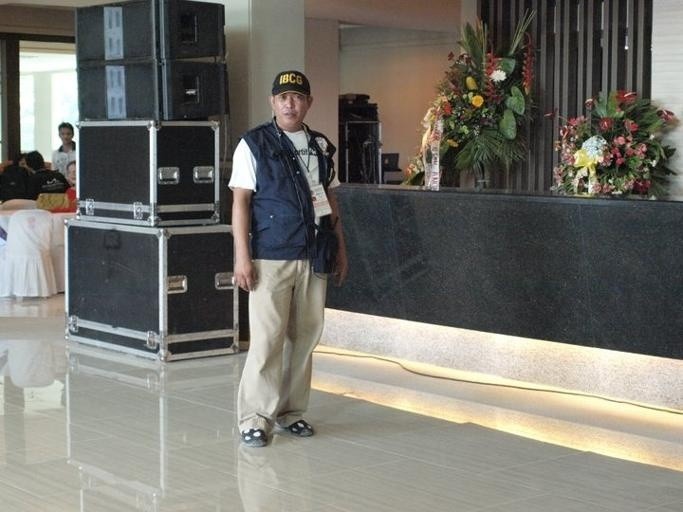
[400, 8, 538, 184]
[545, 90, 678, 201]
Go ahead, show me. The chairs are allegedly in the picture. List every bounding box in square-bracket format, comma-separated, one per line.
[4, 209, 57, 301]
[2, 199, 37, 209]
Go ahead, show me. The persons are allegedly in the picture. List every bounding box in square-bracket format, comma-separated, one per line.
[228, 72, 350, 447]
[0, 122, 77, 208]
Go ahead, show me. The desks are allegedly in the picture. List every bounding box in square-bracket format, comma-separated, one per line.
[0, 212, 77, 292]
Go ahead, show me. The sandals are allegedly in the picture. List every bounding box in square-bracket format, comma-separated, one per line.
[279, 419, 314, 437]
[239, 429, 267, 448]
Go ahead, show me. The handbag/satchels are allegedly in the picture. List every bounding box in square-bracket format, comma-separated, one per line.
[35, 191, 70, 210]
[310, 227, 340, 275]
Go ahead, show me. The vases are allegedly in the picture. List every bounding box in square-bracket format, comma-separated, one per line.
[606, 185, 631, 199]
[474, 165, 504, 187]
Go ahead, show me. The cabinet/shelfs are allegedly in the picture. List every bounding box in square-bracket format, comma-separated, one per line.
[340, 121, 382, 184]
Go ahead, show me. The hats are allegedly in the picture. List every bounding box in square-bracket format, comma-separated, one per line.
[271, 70, 311, 98]
